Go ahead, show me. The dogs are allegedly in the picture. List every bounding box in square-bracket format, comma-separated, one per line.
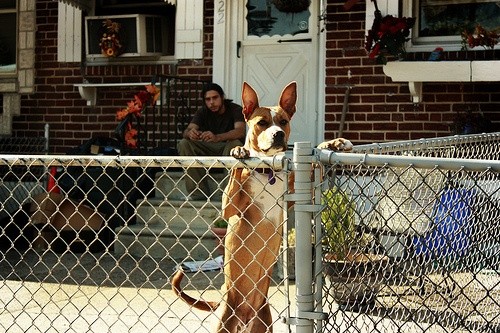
[171, 81, 353, 333]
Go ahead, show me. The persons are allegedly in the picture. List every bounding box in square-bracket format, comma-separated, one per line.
[177, 84, 246, 201]
